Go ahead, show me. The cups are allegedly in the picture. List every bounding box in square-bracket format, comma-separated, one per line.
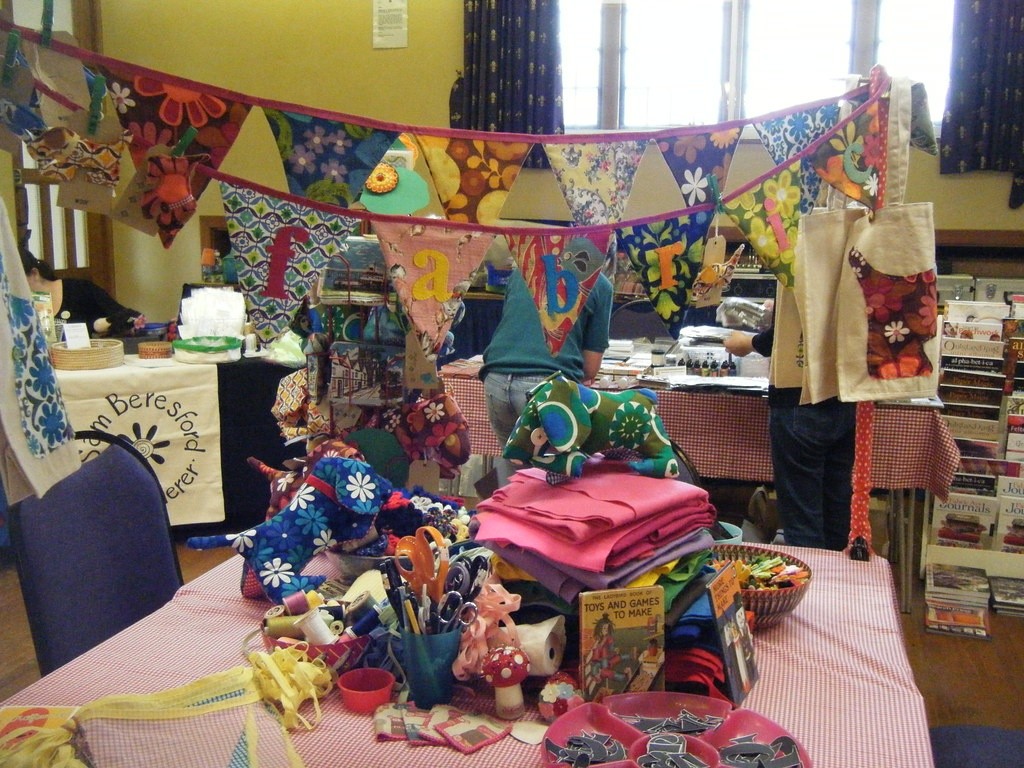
[738, 247, 760, 268]
[202, 248, 215, 266]
[398, 623, 463, 710]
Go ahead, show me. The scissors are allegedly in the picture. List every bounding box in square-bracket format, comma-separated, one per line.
[394, 526, 488, 634]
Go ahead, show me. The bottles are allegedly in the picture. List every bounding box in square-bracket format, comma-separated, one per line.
[600, 375, 611, 388]
[669, 358, 737, 377]
[618, 376, 630, 389]
[650, 349, 665, 376]
[201, 248, 237, 285]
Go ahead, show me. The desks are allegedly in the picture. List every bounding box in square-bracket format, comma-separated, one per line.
[0, 540, 937, 768]
[0, 332, 305, 556]
[440, 353, 961, 612]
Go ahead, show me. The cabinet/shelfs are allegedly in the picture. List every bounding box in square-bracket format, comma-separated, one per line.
[917, 275, 1024, 581]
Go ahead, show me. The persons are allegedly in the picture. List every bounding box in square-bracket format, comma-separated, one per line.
[478, 237, 613, 468]
[20, 248, 136, 338]
[723, 298, 857, 550]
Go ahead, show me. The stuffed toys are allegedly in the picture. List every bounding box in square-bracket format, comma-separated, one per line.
[187, 394, 472, 607]
[502, 371, 680, 479]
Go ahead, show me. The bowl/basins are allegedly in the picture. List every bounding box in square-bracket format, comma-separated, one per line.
[710, 544, 812, 629]
[714, 521, 742, 545]
[336, 667, 395, 712]
[258, 623, 371, 675]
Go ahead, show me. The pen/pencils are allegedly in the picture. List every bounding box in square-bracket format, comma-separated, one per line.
[380, 559, 437, 689]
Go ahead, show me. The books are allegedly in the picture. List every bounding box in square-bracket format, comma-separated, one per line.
[579, 586, 665, 704]
[706, 561, 759, 707]
[923, 275, 1024, 640]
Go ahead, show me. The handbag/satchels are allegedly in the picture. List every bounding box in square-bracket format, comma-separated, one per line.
[768, 77, 939, 405]
